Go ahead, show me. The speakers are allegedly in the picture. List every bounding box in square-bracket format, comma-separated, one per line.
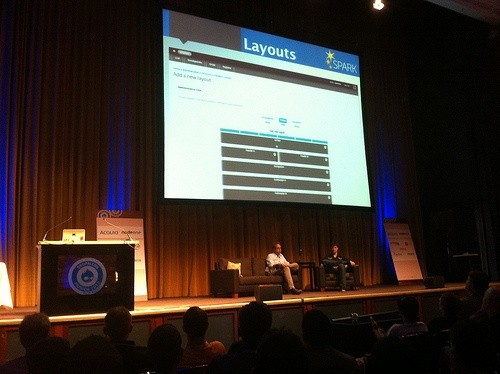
[423, 276, 445, 289]
[254, 285, 282, 301]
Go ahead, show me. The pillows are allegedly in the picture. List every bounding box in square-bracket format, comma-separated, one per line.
[217, 257, 268, 276]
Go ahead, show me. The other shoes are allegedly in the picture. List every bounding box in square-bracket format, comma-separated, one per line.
[291, 288, 302, 294]
[273, 264, 284, 270]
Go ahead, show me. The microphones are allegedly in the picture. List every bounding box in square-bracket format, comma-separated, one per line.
[107, 222, 132, 241]
[43, 217, 72, 241]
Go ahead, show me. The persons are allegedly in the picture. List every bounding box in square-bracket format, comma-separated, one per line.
[0, 270, 500, 374]
[320, 241, 351, 292]
[264, 241, 302, 294]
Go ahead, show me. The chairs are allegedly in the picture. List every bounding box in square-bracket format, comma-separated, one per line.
[179, 310, 500, 374]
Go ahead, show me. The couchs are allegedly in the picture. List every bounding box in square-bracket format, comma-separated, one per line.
[314, 257, 360, 292]
[210, 257, 315, 299]
